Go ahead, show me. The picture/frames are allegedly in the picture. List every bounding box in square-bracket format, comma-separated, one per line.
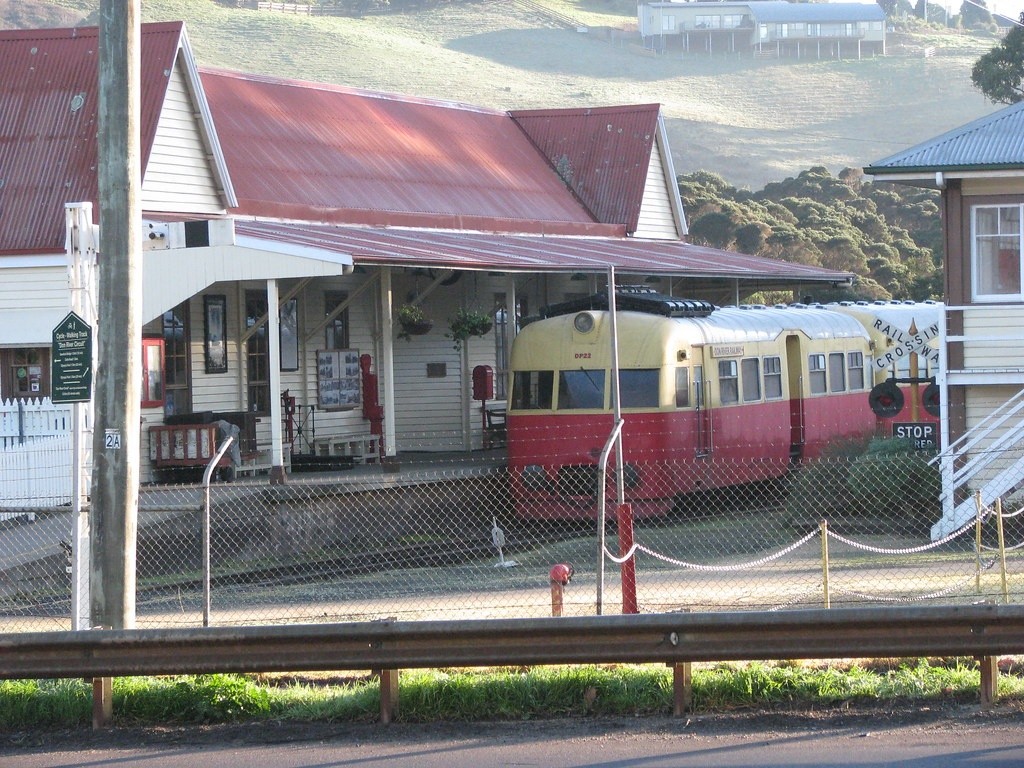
[279, 297, 299, 370]
[204, 295, 229, 374]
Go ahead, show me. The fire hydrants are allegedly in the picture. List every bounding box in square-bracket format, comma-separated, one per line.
[550, 562, 575, 617]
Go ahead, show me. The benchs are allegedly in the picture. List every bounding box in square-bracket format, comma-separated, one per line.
[233, 433, 381, 480]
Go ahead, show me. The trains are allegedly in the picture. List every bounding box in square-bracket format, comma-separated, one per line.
[506, 285, 945, 521]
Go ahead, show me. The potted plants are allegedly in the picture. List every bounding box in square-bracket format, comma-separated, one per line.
[396, 304, 434, 343]
[446, 303, 493, 351]
